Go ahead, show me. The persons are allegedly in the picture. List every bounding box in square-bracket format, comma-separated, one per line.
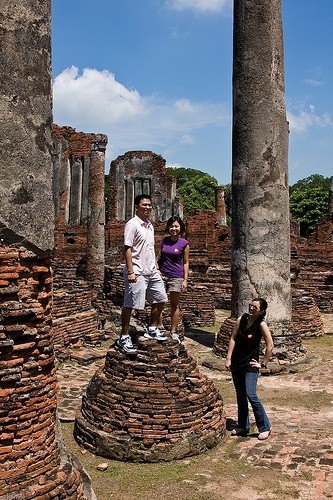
[116, 194, 168, 352]
[225, 297, 274, 440]
[158, 215, 190, 344]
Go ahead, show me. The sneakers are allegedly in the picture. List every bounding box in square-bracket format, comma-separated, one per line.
[143, 324, 168, 341]
[117, 334, 137, 353]
[170, 331, 179, 340]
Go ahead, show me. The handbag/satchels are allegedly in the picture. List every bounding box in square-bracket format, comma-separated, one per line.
[157, 255, 161, 269]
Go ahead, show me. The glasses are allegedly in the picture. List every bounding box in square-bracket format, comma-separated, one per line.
[249, 304, 261, 310]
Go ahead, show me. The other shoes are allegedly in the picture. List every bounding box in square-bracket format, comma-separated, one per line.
[231, 427, 249, 434]
[258, 426, 272, 439]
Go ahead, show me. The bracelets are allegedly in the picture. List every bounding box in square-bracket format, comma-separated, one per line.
[260, 361, 267, 369]
[126, 271, 134, 275]
